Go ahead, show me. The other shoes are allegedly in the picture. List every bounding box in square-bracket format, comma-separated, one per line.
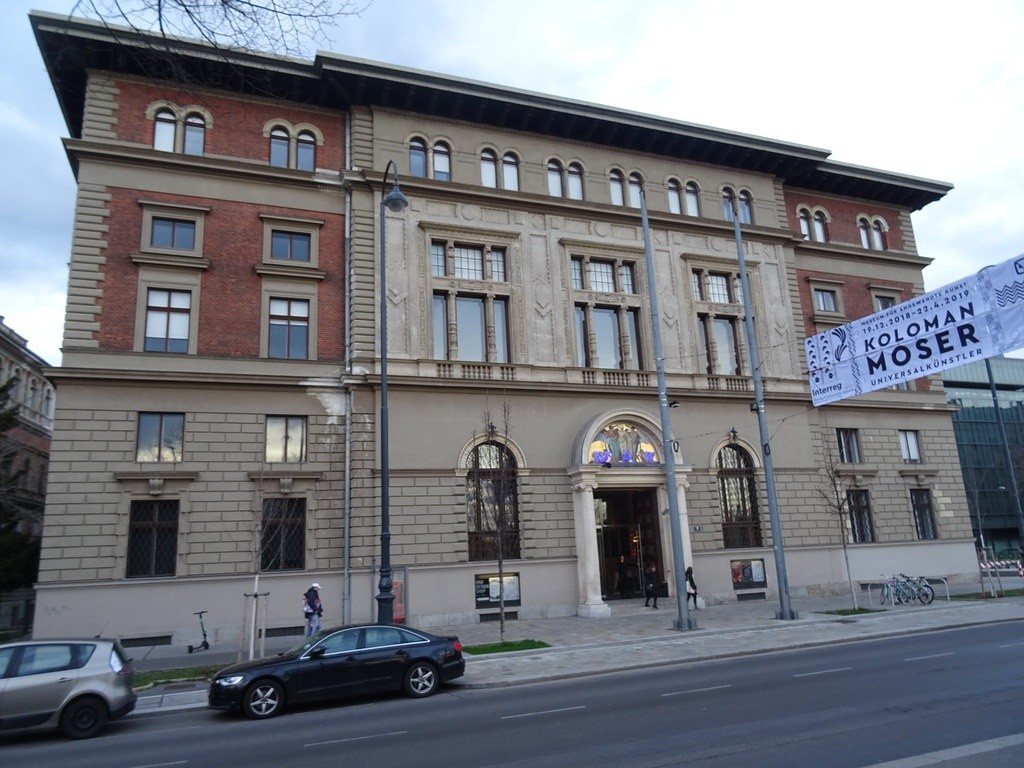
[653, 604, 659, 609]
[645, 604, 650, 607]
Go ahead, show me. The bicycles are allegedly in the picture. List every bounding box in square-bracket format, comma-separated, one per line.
[879, 573, 934, 606]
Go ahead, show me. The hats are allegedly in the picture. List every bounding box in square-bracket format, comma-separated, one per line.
[312, 582, 322, 589]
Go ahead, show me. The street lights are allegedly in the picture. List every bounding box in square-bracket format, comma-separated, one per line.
[373, 158, 410, 628]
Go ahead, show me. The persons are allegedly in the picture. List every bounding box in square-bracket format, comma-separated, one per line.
[686, 567, 698, 609]
[645, 563, 659, 609]
[304, 583, 323, 638]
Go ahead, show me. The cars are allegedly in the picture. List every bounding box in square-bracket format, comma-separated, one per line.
[204, 621, 466, 721]
[0, 636, 139, 740]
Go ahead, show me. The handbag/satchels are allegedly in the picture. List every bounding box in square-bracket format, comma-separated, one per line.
[301, 595, 320, 613]
[686, 574, 696, 594]
